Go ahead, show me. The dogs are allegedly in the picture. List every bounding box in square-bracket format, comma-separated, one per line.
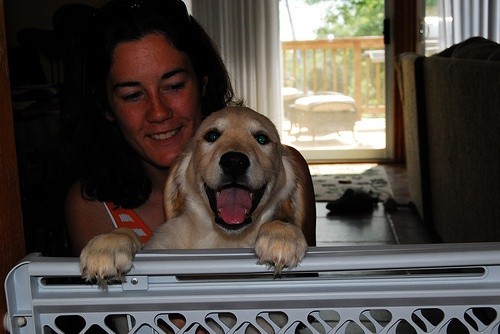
[79, 105, 307, 294]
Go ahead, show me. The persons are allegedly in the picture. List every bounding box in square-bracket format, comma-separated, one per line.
[64, 6, 317, 334]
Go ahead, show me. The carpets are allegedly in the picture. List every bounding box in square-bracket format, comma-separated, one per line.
[311, 164, 414, 205]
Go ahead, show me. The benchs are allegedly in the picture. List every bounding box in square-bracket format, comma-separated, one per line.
[282, 86, 362, 143]
